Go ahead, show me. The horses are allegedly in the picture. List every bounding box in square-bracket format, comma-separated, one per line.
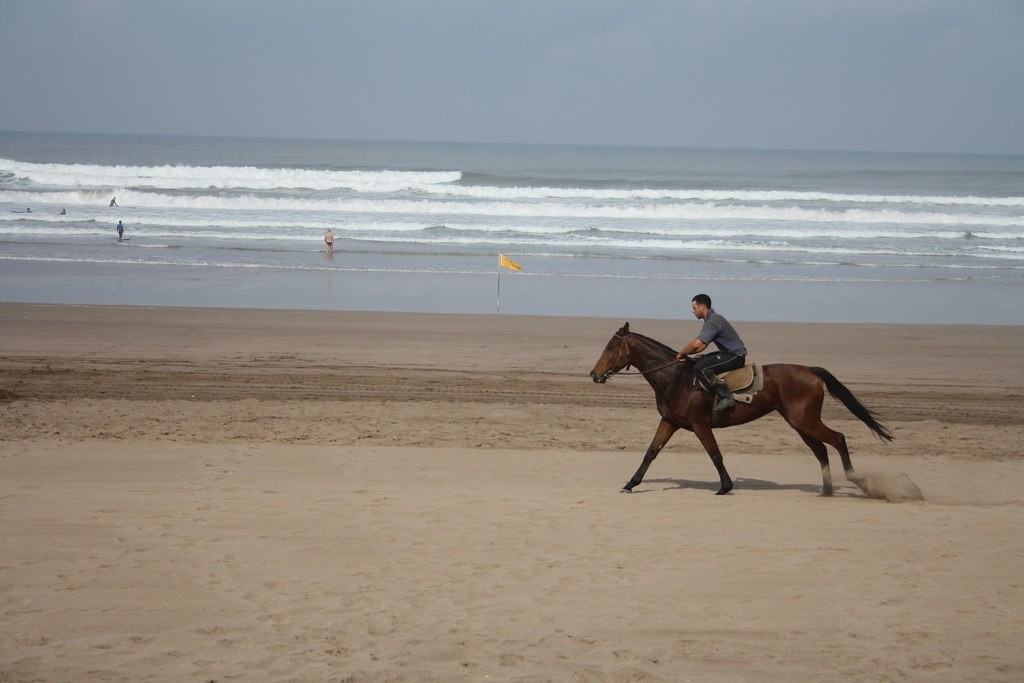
[589, 322, 894, 498]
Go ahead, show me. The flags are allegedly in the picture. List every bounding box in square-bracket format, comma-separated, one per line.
[501, 255, 521, 271]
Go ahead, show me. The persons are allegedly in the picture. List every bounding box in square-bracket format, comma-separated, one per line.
[116, 220, 124, 242]
[26, 208, 30, 212]
[676, 294, 746, 411]
[324, 229, 334, 255]
[110, 197, 117, 207]
[61, 209, 65, 214]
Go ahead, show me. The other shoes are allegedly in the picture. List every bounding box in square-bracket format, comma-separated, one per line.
[713, 398, 735, 412]
